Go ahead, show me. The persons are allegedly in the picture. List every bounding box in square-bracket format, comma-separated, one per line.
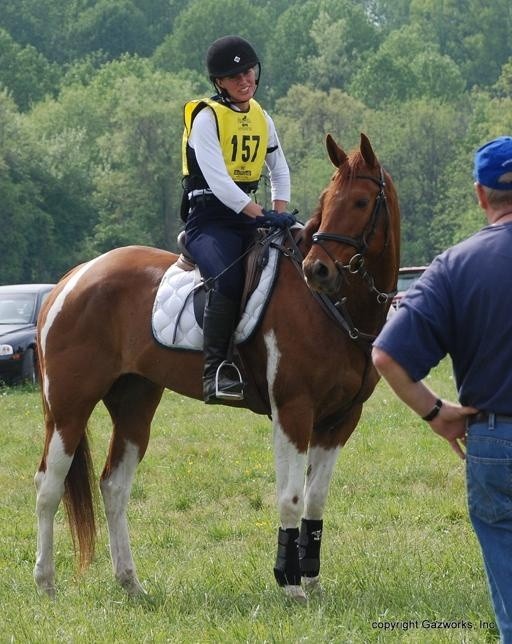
[179, 35, 291, 403]
[372, 136, 512, 644]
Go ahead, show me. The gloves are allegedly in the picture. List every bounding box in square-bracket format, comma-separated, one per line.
[256, 208, 296, 227]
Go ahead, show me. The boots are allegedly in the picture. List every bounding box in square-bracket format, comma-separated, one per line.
[202, 289, 242, 404]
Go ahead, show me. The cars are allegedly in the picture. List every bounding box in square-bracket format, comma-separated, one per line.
[391, 266, 429, 308]
[0, 284, 57, 385]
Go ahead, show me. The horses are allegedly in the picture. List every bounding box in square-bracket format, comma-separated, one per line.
[33, 132, 402, 614]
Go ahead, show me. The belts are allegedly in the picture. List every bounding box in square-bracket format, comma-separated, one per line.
[467, 411, 512, 428]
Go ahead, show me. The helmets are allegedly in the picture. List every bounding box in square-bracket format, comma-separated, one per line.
[207, 36, 258, 82]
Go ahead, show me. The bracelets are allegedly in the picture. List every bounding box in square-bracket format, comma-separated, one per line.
[422, 399, 443, 422]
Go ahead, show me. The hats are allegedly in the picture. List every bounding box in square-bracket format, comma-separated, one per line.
[473, 136, 512, 190]
